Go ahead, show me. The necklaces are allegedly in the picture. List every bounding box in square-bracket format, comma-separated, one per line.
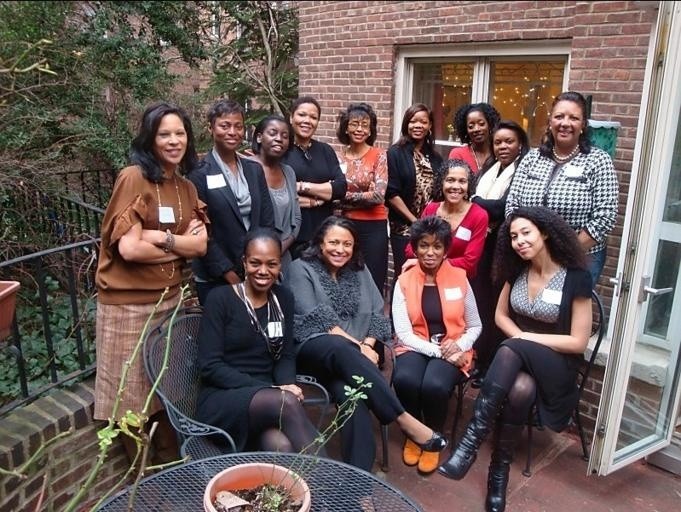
[552, 144, 580, 162]
[235, 283, 286, 361]
[157, 172, 184, 280]
[294, 142, 314, 164]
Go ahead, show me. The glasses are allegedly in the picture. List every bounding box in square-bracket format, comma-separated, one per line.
[300, 145, 312, 163]
[348, 123, 369, 129]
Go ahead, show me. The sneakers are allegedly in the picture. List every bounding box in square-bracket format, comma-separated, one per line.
[403, 439, 421, 466]
[418, 450, 439, 474]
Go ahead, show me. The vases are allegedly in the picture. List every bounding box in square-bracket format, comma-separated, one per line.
[0, 281, 22, 341]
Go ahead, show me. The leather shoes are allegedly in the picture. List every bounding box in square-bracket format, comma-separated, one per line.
[413, 431, 448, 452]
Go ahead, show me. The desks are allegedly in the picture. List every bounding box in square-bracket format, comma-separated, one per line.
[86, 449, 429, 511]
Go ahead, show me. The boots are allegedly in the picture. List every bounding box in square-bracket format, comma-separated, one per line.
[486, 423, 524, 512]
[438, 383, 506, 480]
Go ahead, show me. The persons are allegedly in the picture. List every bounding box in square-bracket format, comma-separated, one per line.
[391, 217, 484, 475]
[506, 91, 620, 292]
[282, 96, 349, 245]
[388, 103, 446, 277]
[448, 102, 503, 174]
[405, 158, 492, 284]
[188, 99, 275, 306]
[435, 205, 593, 512]
[466, 121, 531, 389]
[197, 227, 330, 462]
[93, 102, 209, 482]
[334, 102, 389, 373]
[246, 115, 303, 265]
[281, 216, 449, 512]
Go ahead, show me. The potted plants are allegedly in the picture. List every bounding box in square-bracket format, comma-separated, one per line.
[202, 374, 374, 511]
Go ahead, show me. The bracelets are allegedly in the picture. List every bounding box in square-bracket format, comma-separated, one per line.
[299, 180, 313, 193]
[309, 195, 319, 208]
[161, 229, 178, 255]
[360, 340, 374, 351]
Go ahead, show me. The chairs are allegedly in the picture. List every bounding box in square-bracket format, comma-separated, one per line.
[142, 305, 330, 463]
[523, 289, 606, 476]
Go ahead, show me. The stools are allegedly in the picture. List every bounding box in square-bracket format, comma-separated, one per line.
[446, 369, 474, 456]
[301, 338, 396, 473]
